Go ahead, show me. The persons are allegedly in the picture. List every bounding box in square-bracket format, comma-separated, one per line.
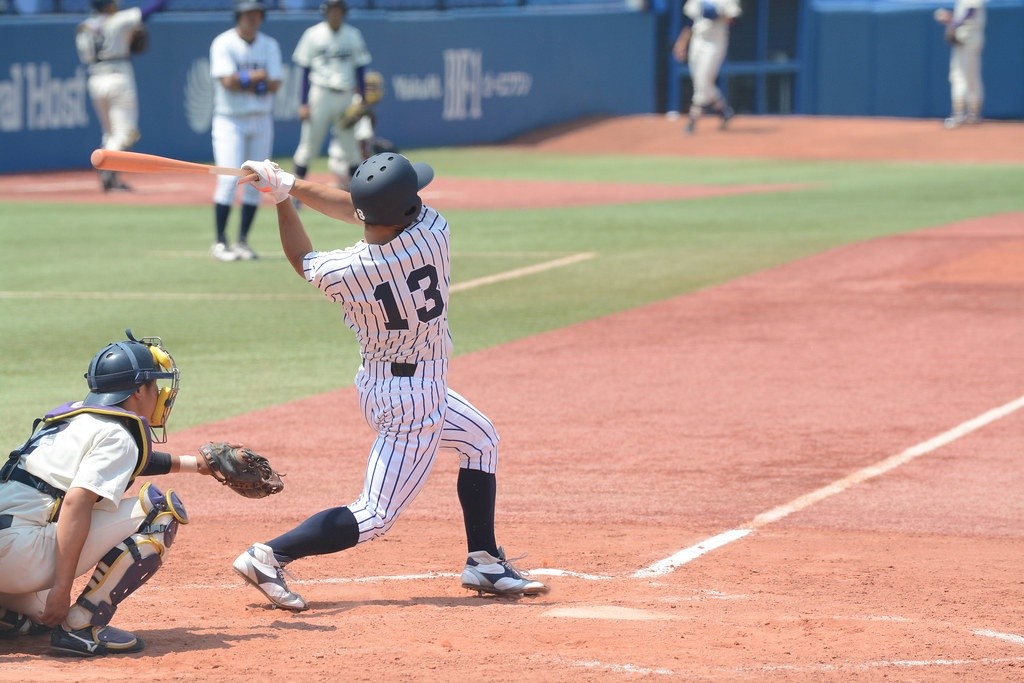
[670, 0, 742, 136]
[327, 70, 398, 193]
[75, 0, 166, 189]
[231, 152, 552, 612]
[932, 0, 987, 130]
[291, 1, 372, 210]
[209, 0, 283, 264]
[0, 331, 288, 658]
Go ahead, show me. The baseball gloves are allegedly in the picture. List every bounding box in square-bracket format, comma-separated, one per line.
[130, 27, 147, 52]
[198, 442, 284, 499]
[345, 104, 377, 131]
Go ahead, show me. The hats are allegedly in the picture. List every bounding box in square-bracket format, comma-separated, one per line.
[82, 341, 162, 407]
[235, 0, 267, 12]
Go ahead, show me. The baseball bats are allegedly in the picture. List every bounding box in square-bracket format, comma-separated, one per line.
[89, 148, 258, 185]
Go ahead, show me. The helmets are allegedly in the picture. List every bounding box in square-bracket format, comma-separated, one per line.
[350, 153, 434, 224]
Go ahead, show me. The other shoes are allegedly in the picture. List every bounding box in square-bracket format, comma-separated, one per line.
[966, 112, 979, 124]
[101, 170, 129, 191]
[686, 122, 693, 134]
[719, 106, 735, 130]
[944, 114, 964, 129]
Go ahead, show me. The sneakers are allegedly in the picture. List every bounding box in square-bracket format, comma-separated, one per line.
[50, 621, 144, 658]
[213, 242, 240, 262]
[234, 241, 257, 260]
[461, 545, 549, 598]
[233, 543, 308, 611]
[1, 608, 55, 639]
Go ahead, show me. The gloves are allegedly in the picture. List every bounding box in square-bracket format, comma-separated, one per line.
[240, 159, 296, 205]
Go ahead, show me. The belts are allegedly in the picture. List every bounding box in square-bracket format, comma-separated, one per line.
[362, 358, 416, 377]
[0, 515, 13, 530]
